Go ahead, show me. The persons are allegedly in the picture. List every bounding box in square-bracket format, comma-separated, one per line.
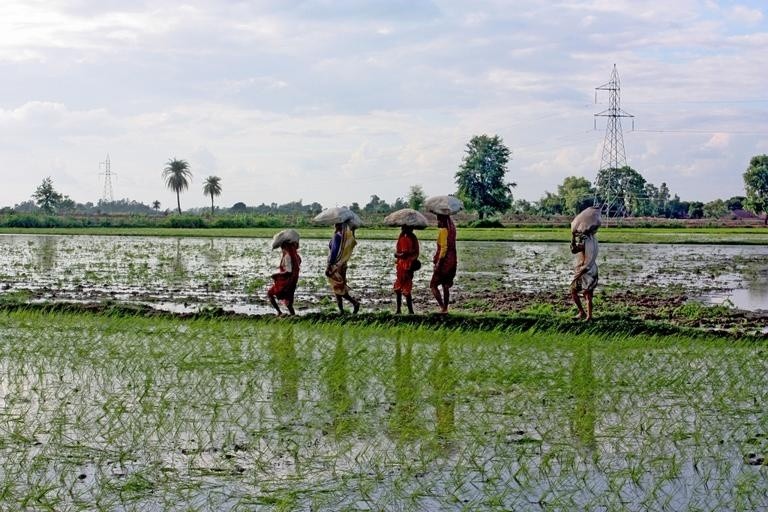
[393, 225, 419, 315]
[267, 240, 299, 317]
[428, 212, 457, 315]
[325, 219, 360, 317]
[570, 225, 598, 322]
